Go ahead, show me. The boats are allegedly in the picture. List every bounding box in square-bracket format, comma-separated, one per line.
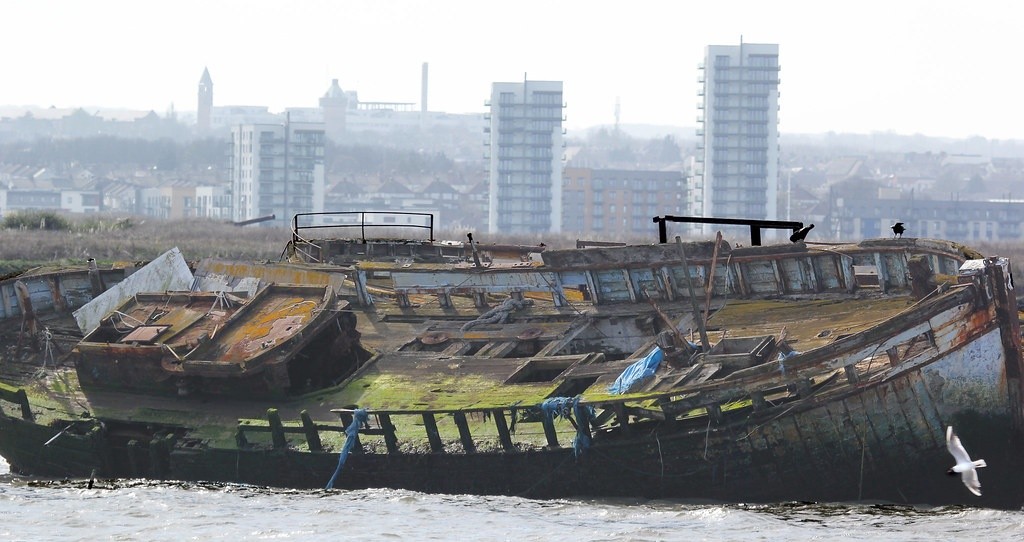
[1, 209, 1024, 513]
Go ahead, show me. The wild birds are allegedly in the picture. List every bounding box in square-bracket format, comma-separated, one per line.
[890, 222, 907, 238]
[944, 425, 988, 497]
[790, 224, 814, 243]
